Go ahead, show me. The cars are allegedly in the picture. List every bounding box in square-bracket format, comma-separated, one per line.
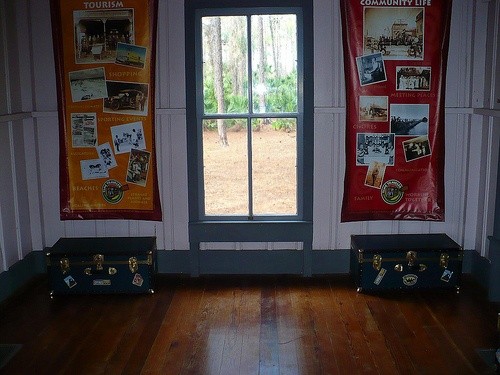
[105, 88, 143, 111]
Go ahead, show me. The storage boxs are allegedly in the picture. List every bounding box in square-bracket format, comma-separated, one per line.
[43, 236, 160, 296]
[350, 232, 464, 301]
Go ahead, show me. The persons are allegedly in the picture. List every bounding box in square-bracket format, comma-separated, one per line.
[371, 168, 379, 186]
[130, 129, 139, 148]
[114, 134, 120, 152]
[399, 75, 426, 89]
[81, 34, 130, 52]
[135, 93, 145, 111]
[100, 148, 111, 166]
[357, 141, 394, 164]
